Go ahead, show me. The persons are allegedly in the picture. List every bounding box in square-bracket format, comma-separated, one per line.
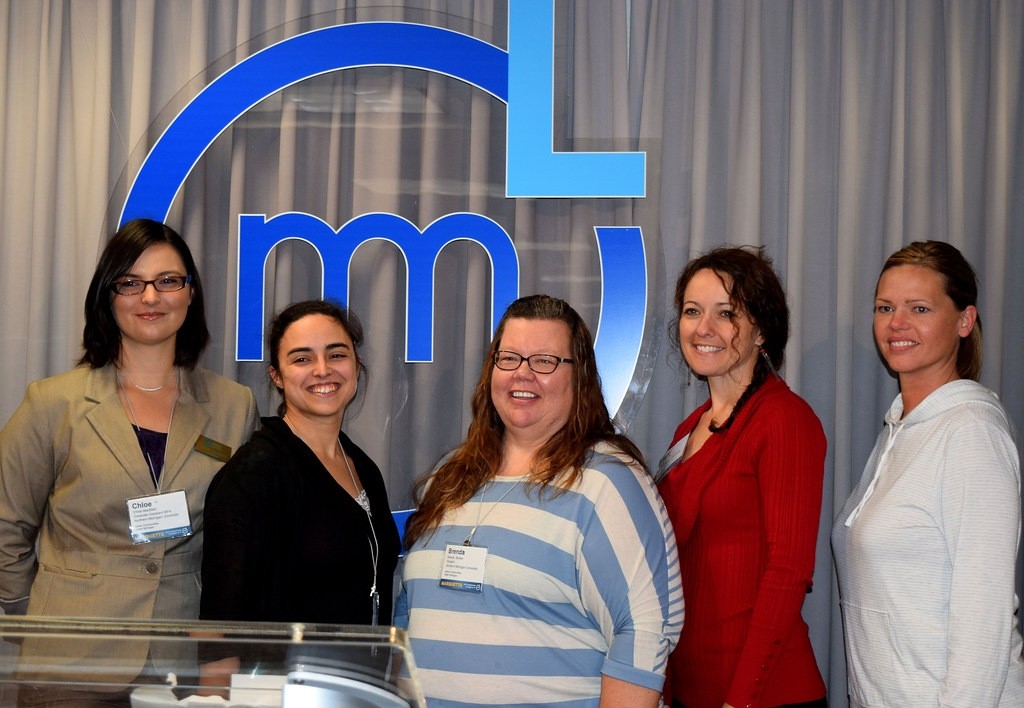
[195, 300, 406, 707]
[391, 289, 690, 708]
[650, 242, 830, 708]
[0, 217, 260, 705]
[830, 238, 1024, 707]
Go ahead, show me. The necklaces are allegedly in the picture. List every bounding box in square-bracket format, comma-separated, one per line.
[113, 351, 180, 393]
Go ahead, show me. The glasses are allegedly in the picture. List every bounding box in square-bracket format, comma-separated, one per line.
[112, 274, 192, 296]
[492, 351, 574, 374]
